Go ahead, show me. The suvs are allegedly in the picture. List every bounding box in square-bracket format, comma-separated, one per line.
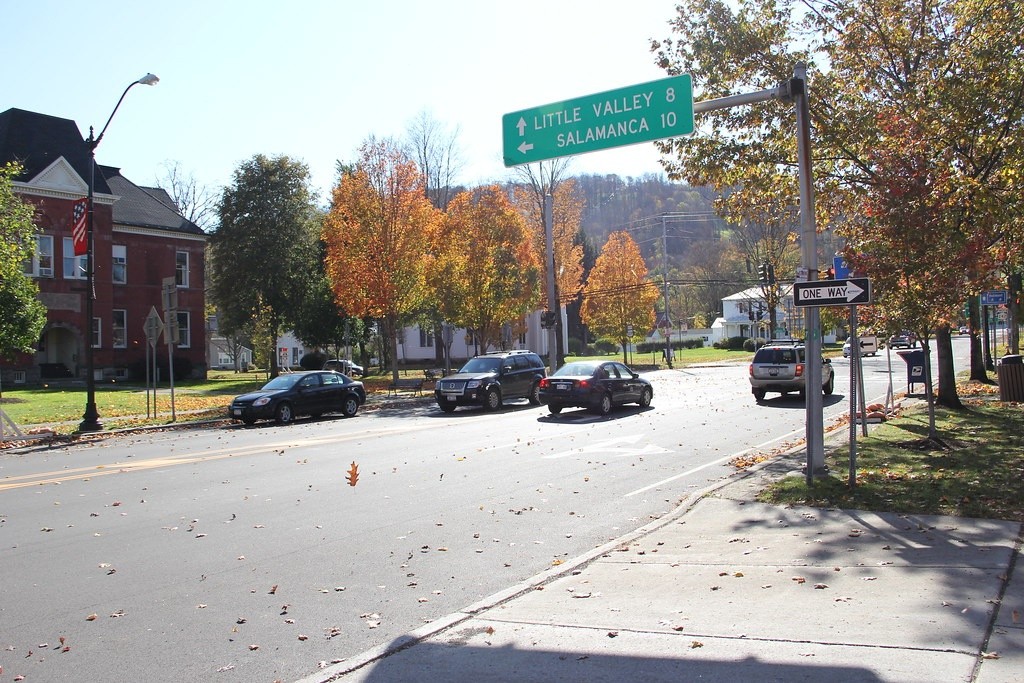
[435, 350, 548, 412]
[749, 340, 835, 401]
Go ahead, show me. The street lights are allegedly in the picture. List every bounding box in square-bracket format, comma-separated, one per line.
[77, 71, 159, 432]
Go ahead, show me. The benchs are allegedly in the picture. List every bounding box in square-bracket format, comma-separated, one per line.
[388, 378, 424, 399]
[423, 367, 443, 380]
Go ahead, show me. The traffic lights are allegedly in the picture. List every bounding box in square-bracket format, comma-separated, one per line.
[541, 310, 548, 329]
[765, 264, 775, 284]
[757, 264, 767, 285]
[547, 310, 556, 329]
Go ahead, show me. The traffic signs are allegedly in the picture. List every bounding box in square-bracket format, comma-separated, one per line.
[793, 277, 871, 309]
[502, 72, 695, 169]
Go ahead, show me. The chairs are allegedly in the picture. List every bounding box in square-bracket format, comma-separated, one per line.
[330, 377, 340, 385]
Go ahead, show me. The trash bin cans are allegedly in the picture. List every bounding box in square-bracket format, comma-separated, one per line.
[997, 354, 1024, 402]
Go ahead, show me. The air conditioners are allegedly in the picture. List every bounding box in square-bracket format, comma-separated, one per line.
[40, 268, 53, 277]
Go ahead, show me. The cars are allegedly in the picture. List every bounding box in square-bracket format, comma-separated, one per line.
[322, 360, 364, 376]
[538, 361, 653, 416]
[227, 370, 367, 427]
[959, 326, 971, 336]
[843, 336, 875, 358]
[888, 330, 916, 350]
